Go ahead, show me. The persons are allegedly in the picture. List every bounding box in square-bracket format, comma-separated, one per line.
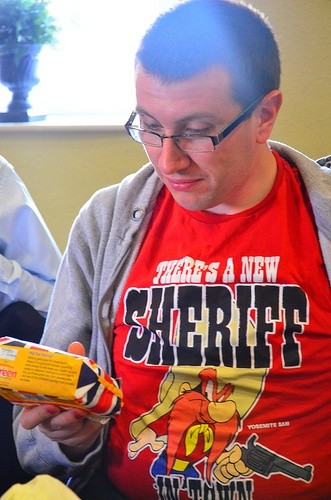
[12, 0, 331, 500]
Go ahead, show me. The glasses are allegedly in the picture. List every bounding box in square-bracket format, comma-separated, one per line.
[124, 88, 273, 152]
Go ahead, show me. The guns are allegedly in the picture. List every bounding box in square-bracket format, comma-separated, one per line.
[239, 433, 314, 484]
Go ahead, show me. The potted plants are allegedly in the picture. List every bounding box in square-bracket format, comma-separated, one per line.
[0, 0, 61, 123]
